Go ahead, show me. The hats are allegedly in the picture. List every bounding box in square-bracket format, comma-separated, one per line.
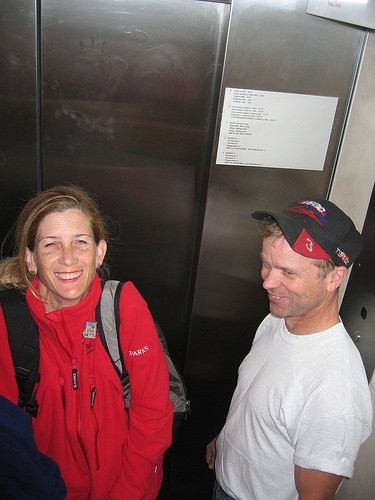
[252, 198, 365, 269]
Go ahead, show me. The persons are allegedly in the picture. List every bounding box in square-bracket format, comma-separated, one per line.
[205, 198, 373, 500]
[0, 186, 174, 500]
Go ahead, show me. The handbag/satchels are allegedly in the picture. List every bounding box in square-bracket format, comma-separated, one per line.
[96, 279, 189, 433]
